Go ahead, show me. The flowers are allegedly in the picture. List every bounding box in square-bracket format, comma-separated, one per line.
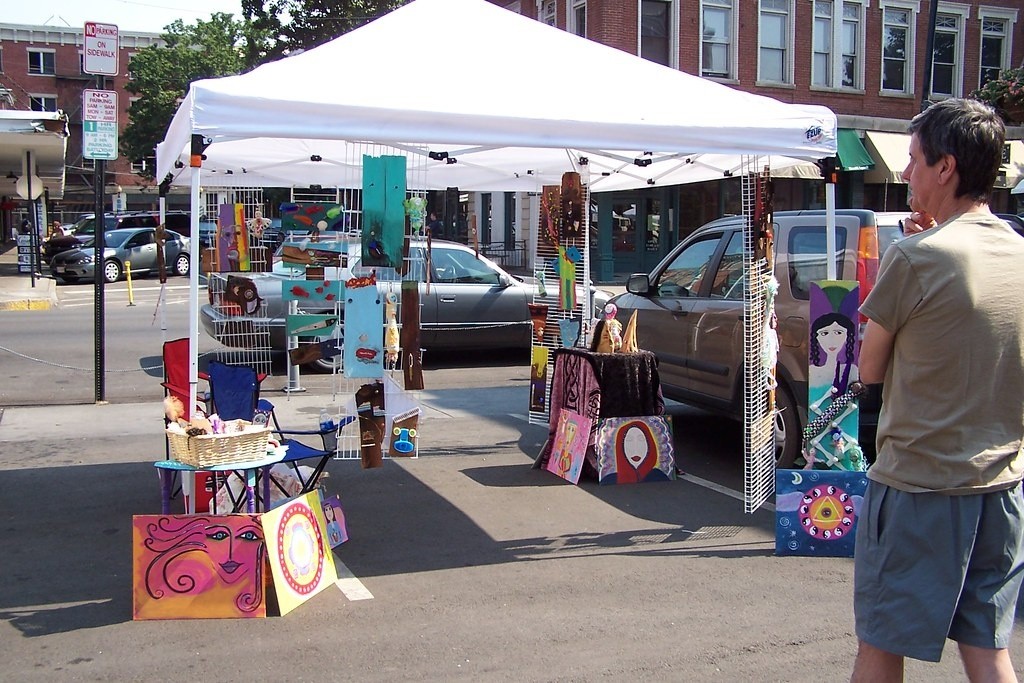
[969, 65, 1024, 103]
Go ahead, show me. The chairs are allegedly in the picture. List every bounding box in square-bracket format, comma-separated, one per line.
[159, 337, 267, 500]
[205, 359, 356, 512]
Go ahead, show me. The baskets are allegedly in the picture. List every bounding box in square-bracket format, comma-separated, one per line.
[164, 426, 275, 467]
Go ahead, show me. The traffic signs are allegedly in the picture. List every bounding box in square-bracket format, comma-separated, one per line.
[82, 89, 119, 160]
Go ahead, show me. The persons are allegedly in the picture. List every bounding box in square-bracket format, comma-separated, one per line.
[51, 221, 64, 238]
[850, 99, 1024, 683]
[16, 214, 31, 235]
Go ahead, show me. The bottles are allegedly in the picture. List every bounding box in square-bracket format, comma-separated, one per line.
[319, 409, 336, 451]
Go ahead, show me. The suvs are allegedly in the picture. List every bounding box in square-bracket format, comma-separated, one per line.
[41, 210, 191, 265]
[597, 209, 1024, 469]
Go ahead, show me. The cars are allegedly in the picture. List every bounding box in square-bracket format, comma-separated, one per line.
[49, 227, 191, 282]
[200, 236, 615, 374]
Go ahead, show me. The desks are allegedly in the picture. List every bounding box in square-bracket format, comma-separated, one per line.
[153, 448, 286, 513]
[539, 345, 666, 474]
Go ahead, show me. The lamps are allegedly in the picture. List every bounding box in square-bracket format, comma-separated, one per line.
[6, 171, 18, 184]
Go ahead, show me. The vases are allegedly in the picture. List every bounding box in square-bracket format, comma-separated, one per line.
[999, 102, 1024, 121]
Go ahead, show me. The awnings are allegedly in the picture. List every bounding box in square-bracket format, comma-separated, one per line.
[864, 131, 1024, 188]
[836, 127, 875, 171]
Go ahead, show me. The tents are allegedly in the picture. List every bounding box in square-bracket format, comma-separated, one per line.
[157, 0, 838, 514]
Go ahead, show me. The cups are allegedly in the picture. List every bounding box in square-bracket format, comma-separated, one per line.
[559, 318, 580, 346]
